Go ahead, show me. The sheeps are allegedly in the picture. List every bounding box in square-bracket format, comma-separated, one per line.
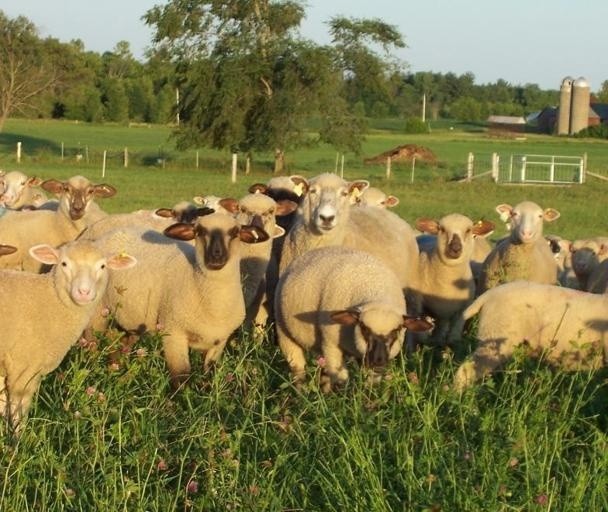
[449, 280, 607, 395]
[73, 210, 270, 391]
[407, 212, 496, 351]
[0, 241, 137, 429]
[279, 171, 421, 352]
[273, 245, 435, 396]
[1, 171, 608, 294]
